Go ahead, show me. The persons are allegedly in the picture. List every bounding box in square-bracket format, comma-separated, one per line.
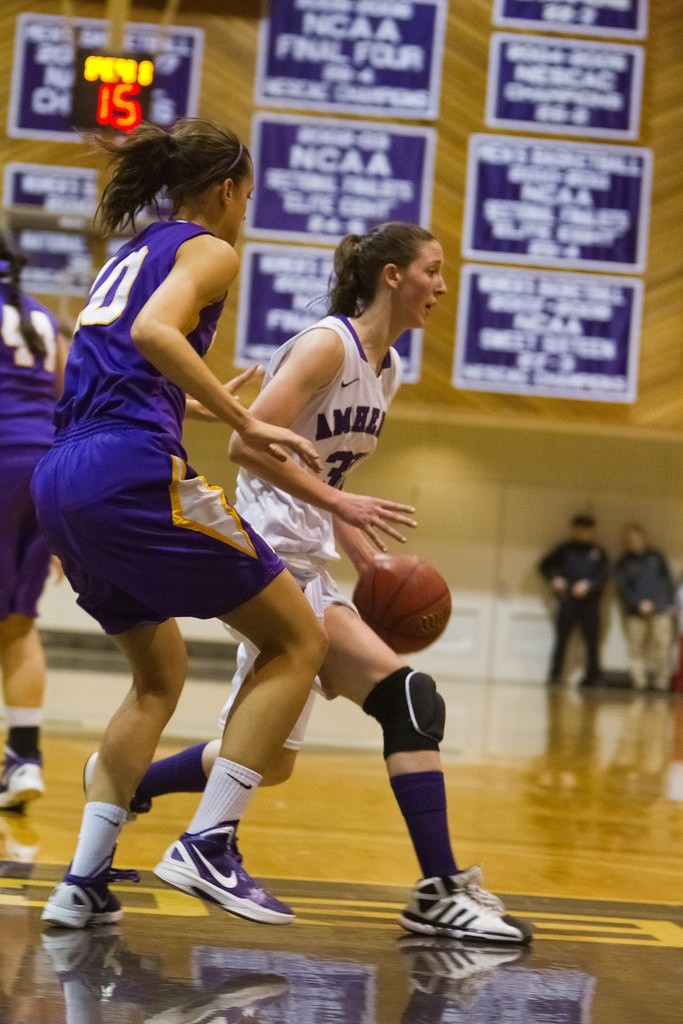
[539, 515, 611, 686]
[1, 223, 66, 810]
[533, 686, 683, 851]
[32, 119, 323, 932]
[612, 523, 675, 690]
[82, 221, 531, 944]
[1, 811, 532, 1023]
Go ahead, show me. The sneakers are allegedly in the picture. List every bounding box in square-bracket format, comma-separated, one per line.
[39, 855, 140, 929]
[141, 972, 289, 1023]
[40, 924, 124, 993]
[397, 863, 533, 946]
[152, 824, 296, 925]
[83, 752, 154, 866]
[397, 934, 530, 1001]
[0, 741, 45, 809]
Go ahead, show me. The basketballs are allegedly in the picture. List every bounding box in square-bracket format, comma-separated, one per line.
[353, 553, 452, 655]
